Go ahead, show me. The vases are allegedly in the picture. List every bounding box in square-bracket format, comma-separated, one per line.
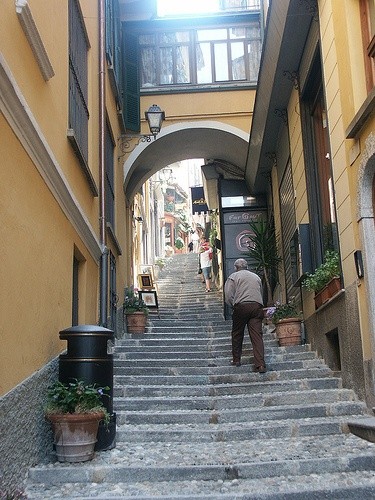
[45, 413, 104, 461]
[276, 318, 302, 346]
[125, 313, 146, 333]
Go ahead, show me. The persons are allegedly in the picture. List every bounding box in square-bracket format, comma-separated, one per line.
[198, 246, 213, 292]
[188, 241, 193, 253]
[224, 258, 267, 373]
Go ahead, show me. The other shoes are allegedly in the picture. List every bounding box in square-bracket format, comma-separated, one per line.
[252, 365, 266, 372]
[230, 360, 241, 366]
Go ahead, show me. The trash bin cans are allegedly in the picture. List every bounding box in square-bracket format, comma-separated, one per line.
[56, 324, 119, 452]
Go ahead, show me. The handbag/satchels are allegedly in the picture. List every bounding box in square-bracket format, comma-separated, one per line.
[209, 252, 213, 259]
[197, 268, 202, 274]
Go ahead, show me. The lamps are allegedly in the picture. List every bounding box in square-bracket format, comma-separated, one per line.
[149, 166, 172, 196]
[118, 104, 166, 159]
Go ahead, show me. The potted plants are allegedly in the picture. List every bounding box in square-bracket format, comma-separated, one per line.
[244, 214, 283, 325]
[301, 251, 344, 308]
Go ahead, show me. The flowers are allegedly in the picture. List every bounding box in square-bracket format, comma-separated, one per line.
[122, 285, 147, 312]
[41, 377, 115, 433]
[272, 300, 305, 324]
